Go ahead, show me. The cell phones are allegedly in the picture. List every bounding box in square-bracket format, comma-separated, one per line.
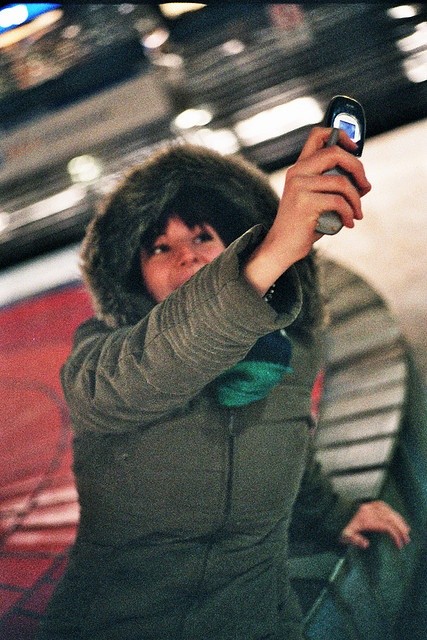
[314, 93, 366, 236]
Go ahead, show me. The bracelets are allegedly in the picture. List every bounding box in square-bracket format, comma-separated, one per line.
[259, 280, 278, 304]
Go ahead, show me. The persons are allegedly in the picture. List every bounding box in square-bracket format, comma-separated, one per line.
[39, 123, 412, 640]
